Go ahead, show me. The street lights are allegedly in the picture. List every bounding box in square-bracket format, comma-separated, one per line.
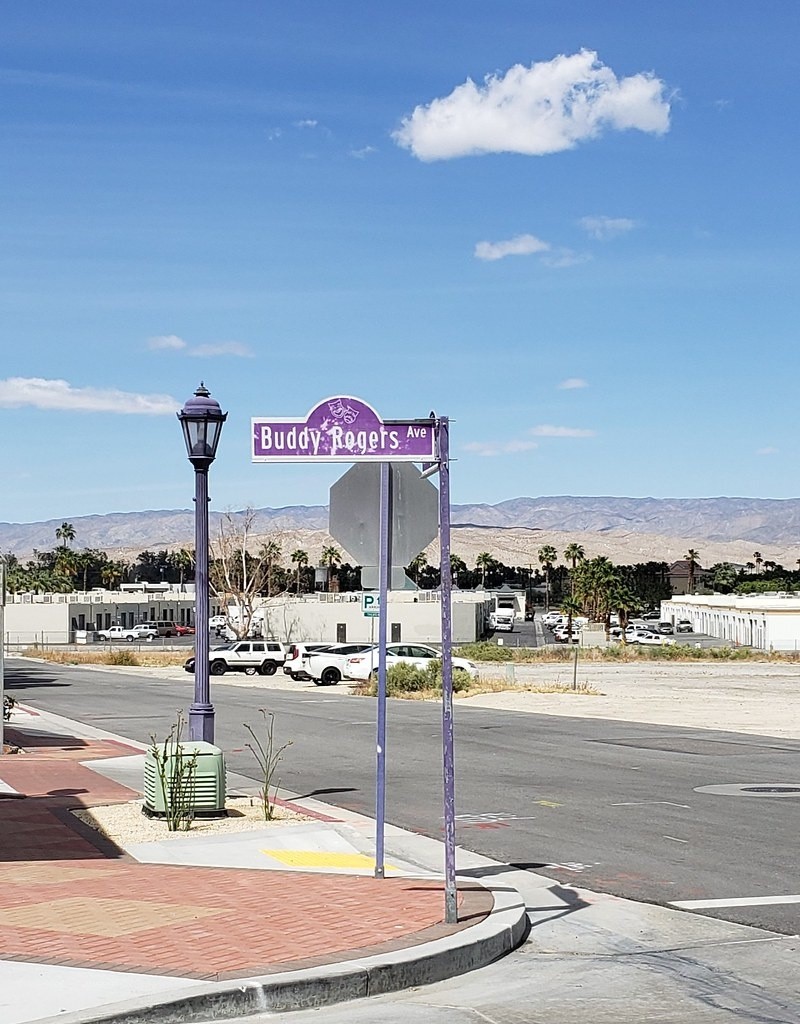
[175, 381, 230, 745]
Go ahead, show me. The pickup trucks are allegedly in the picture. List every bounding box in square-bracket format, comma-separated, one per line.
[133, 624, 159, 640]
[93, 626, 140, 642]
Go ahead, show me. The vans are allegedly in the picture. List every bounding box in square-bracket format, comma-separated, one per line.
[143, 619, 177, 638]
[283, 641, 342, 682]
[494, 615, 514, 631]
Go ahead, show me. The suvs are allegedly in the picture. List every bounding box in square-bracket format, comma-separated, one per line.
[209, 640, 287, 677]
[642, 612, 661, 621]
[657, 622, 673, 635]
[676, 619, 694, 632]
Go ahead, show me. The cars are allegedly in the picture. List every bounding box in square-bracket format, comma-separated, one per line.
[209, 613, 264, 641]
[619, 631, 654, 646]
[302, 640, 399, 686]
[343, 641, 481, 693]
[6, 585, 143, 596]
[171, 621, 191, 637]
[184, 644, 257, 676]
[542, 609, 590, 644]
[256, 589, 381, 604]
[605, 612, 648, 637]
[186, 625, 196, 634]
[639, 634, 677, 647]
